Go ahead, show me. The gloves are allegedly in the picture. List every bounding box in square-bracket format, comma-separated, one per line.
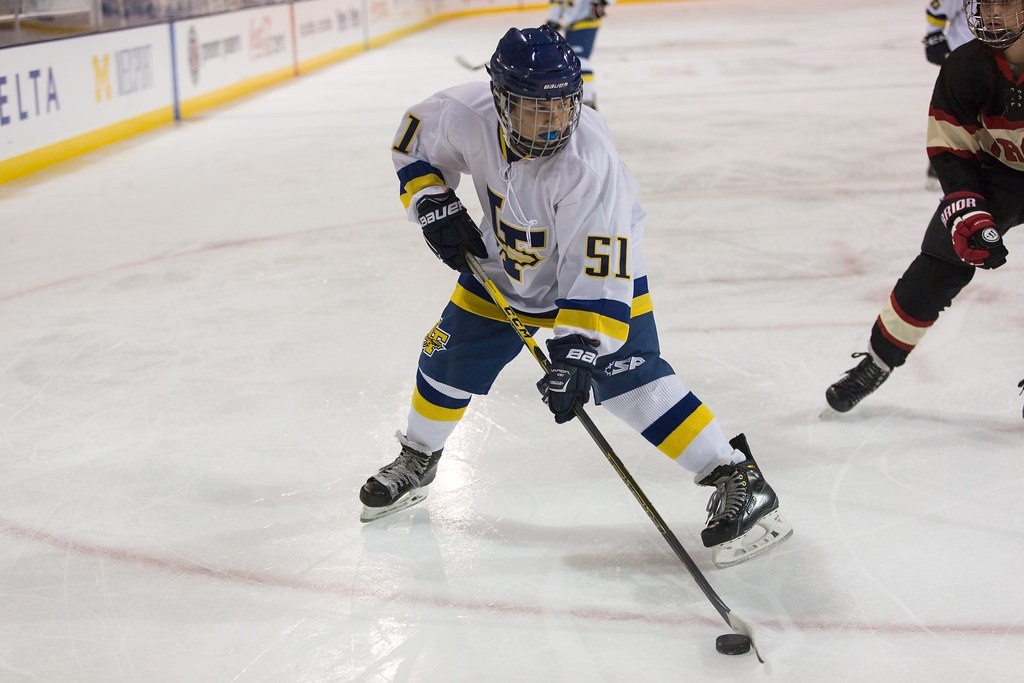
[938, 190, 1009, 270]
[415, 187, 488, 275]
[924, 31, 951, 63]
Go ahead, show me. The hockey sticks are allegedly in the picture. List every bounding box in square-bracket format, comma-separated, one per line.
[464, 250, 771, 664]
[455, 54, 490, 72]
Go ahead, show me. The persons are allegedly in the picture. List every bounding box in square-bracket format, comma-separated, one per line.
[548, 0, 609, 59]
[360, 26, 793, 567]
[921, 0, 977, 191]
[821, 0, 1024, 416]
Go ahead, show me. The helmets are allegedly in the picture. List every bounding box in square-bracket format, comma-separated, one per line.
[489, 23, 584, 155]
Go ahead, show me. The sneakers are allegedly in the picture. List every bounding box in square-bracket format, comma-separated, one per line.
[826, 351, 890, 411]
[538, 333, 601, 423]
[358, 430, 444, 522]
[696, 432, 793, 569]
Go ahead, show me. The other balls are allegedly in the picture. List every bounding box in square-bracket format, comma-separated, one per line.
[715, 632, 752, 656]
